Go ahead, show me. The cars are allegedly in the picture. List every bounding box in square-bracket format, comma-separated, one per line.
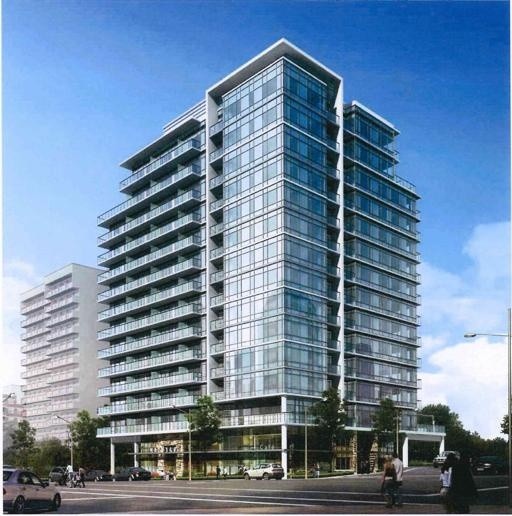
[3, 467, 63, 513]
[114, 466, 153, 481]
[471, 455, 507, 475]
[84, 470, 113, 482]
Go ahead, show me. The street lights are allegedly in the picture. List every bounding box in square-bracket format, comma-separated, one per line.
[54, 415, 75, 471]
[3, 390, 14, 406]
[464, 307, 512, 507]
[171, 404, 192, 481]
[303, 396, 328, 480]
[396, 406, 420, 457]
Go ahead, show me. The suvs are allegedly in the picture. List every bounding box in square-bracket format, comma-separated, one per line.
[241, 462, 285, 480]
[48, 465, 83, 482]
[433, 450, 460, 468]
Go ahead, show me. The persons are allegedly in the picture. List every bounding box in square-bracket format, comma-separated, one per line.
[64, 462, 76, 488]
[77, 465, 86, 488]
[448, 449, 479, 514]
[312, 462, 320, 477]
[223, 466, 227, 480]
[391, 452, 403, 508]
[438, 453, 455, 514]
[216, 465, 221, 480]
[160, 470, 165, 480]
[380, 455, 397, 511]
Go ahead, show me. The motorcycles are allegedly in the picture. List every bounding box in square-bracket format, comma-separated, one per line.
[62, 472, 82, 487]
